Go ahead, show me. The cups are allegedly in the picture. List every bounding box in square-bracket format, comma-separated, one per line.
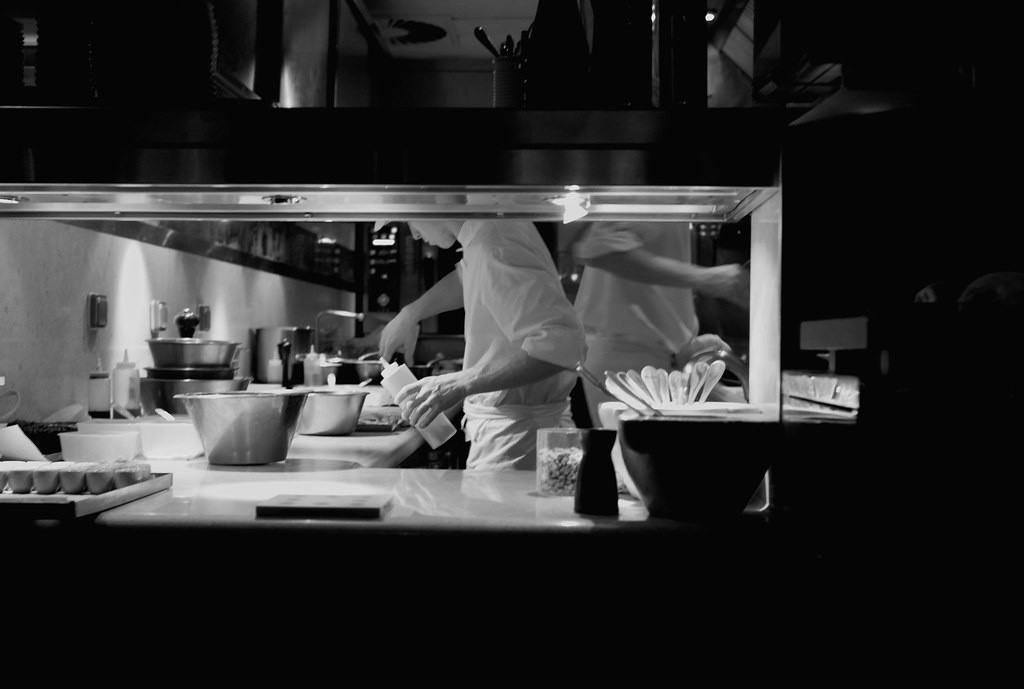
[536, 428, 590, 496]
[0, 460, 152, 498]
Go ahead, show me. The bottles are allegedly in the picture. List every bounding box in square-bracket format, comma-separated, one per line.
[304, 344, 322, 387]
[112, 348, 141, 419]
[88, 357, 111, 419]
[572, 428, 621, 517]
[377, 357, 457, 450]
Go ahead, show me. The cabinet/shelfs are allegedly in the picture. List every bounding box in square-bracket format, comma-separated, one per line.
[0, 0, 782, 689]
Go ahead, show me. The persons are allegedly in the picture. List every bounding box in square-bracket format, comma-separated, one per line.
[373, 218, 590, 470]
[571, 221, 751, 430]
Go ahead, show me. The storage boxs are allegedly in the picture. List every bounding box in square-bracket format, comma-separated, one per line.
[58, 418, 203, 461]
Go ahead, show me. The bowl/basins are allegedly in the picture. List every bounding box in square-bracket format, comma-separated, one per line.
[139, 368, 254, 417]
[295, 355, 383, 386]
[294, 389, 370, 436]
[618, 411, 781, 517]
[172, 390, 315, 465]
[143, 338, 242, 368]
